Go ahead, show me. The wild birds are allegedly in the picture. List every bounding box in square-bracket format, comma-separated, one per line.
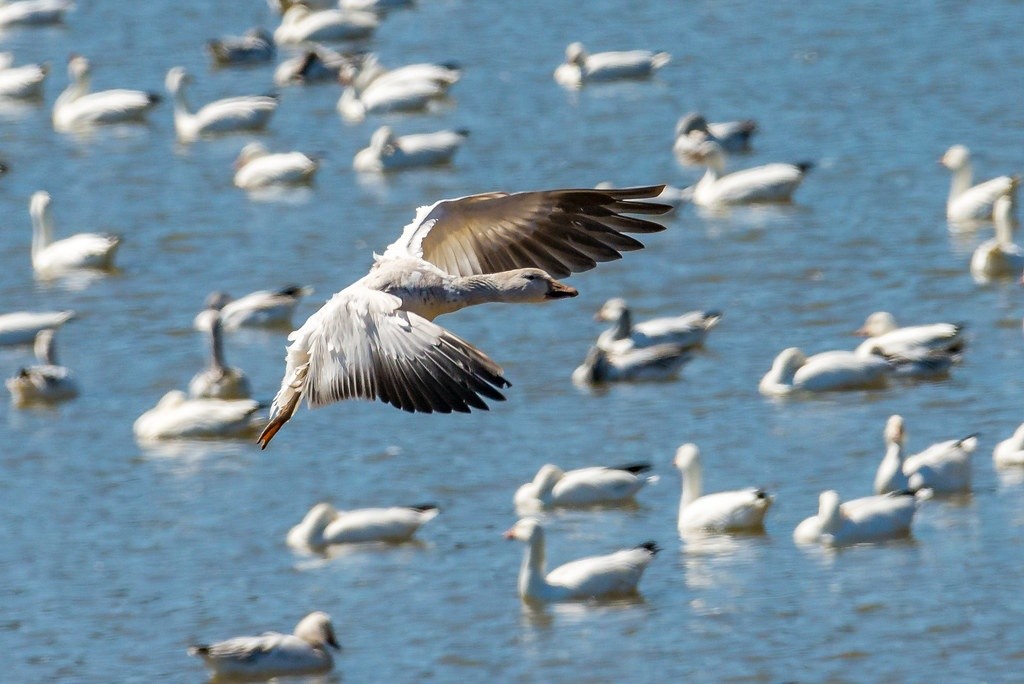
[7, 328, 74, 404]
[759, 346, 893, 398]
[0, 0, 464, 268]
[284, 502, 440, 555]
[671, 442, 772, 534]
[875, 415, 981, 496]
[0, 309, 74, 344]
[188, 316, 247, 400]
[253, 183, 670, 448]
[133, 389, 262, 439]
[183, 610, 340, 677]
[500, 515, 659, 605]
[994, 424, 1024, 465]
[853, 314, 966, 381]
[934, 144, 1024, 279]
[195, 285, 310, 331]
[573, 294, 719, 393]
[793, 484, 932, 548]
[510, 462, 659, 512]
[555, 42, 812, 216]
